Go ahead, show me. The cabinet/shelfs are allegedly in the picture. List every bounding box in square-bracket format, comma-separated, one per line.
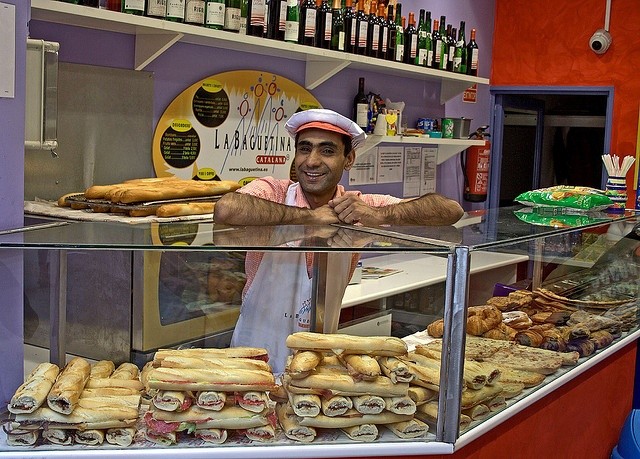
[28, 2, 489, 166]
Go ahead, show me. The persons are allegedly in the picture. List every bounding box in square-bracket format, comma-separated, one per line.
[213, 109, 464, 374]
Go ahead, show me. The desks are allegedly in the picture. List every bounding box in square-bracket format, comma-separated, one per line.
[337, 250, 529, 337]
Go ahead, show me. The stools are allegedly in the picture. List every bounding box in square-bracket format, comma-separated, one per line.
[610, 409, 640, 459]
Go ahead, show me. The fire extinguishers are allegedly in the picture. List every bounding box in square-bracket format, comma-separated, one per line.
[461, 126, 492, 203]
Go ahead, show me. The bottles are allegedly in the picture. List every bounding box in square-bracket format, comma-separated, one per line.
[401, 16, 407, 32]
[369, 1, 379, 58]
[387, 4, 396, 61]
[122, 1, 146, 15]
[301, 0, 316, 46]
[248, 0, 264, 37]
[264, 0, 271, 39]
[333, 7, 346, 51]
[433, 20, 440, 68]
[225, 1, 241, 32]
[240, 0, 247, 35]
[167, 1, 185, 23]
[271, 0, 287, 41]
[447, 24, 455, 70]
[404, 11, 417, 63]
[455, 20, 467, 72]
[346, 0, 357, 53]
[185, 1, 205, 26]
[354, 77, 368, 133]
[357, 0, 368, 56]
[317, 0, 332, 49]
[206, 1, 225, 30]
[147, 1, 167, 18]
[452, 28, 456, 40]
[284, 0, 299, 44]
[439, 15, 447, 69]
[395, 3, 405, 61]
[467, 28, 478, 75]
[379, 4, 388, 59]
[416, 9, 426, 66]
[426, 11, 432, 66]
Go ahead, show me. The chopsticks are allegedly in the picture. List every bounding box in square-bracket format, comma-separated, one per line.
[600, 153, 636, 178]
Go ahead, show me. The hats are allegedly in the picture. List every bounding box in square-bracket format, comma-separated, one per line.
[283, 109, 366, 138]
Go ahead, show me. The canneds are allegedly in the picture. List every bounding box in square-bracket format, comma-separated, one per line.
[442, 118, 455, 139]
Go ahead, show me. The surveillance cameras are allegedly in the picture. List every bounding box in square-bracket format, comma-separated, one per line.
[586, 29, 613, 55]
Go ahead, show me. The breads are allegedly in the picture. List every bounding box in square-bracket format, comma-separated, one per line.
[2, 358, 142, 447]
[396, 337, 579, 432]
[427, 289, 640, 358]
[57, 161, 242, 217]
[144, 347, 280, 446]
[269, 331, 429, 442]
